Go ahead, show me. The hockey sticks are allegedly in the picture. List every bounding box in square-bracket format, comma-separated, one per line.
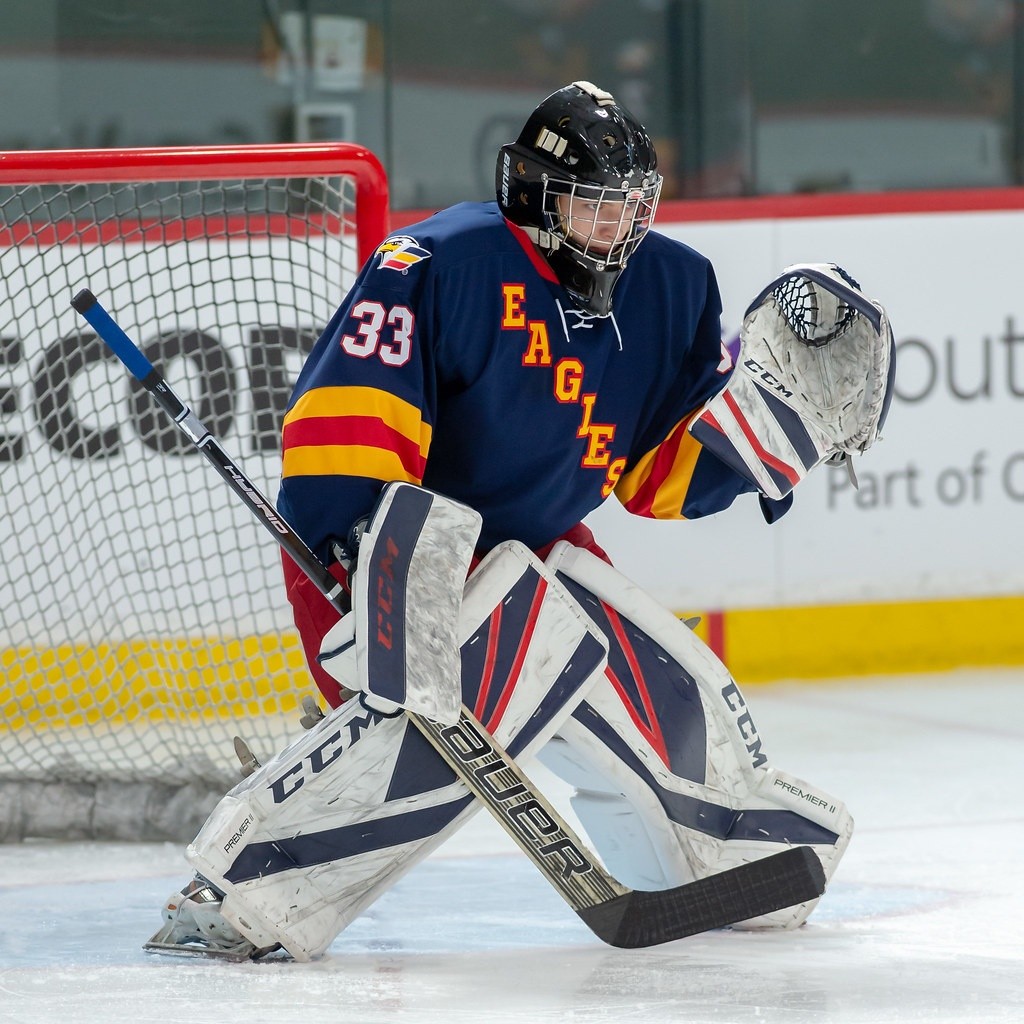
[69, 284, 830, 950]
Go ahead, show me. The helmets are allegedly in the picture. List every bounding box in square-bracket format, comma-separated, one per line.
[494, 80, 665, 318]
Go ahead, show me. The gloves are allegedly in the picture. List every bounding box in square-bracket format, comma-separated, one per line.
[686, 258, 897, 504]
[317, 480, 483, 733]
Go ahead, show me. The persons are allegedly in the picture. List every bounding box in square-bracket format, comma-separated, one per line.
[141, 81, 855, 959]
